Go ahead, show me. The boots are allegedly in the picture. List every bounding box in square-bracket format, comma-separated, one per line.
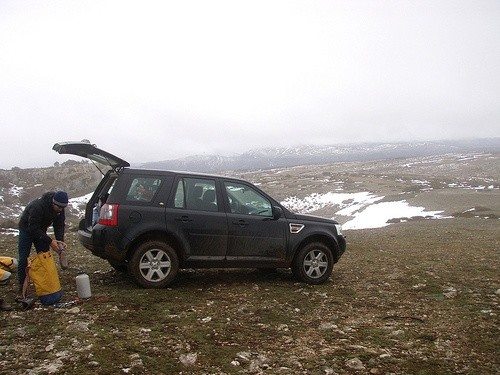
[15, 280, 25, 301]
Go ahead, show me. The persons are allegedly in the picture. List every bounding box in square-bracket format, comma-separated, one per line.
[15, 191, 68, 303]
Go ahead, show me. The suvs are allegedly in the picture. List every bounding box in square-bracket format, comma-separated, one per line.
[52, 138, 347, 289]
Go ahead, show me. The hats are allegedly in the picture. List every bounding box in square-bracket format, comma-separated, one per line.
[52, 191, 68, 207]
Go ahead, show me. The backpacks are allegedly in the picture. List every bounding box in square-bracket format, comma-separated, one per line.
[27, 250, 65, 307]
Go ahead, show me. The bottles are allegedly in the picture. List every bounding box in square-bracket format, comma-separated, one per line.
[58, 241, 69, 269]
[75, 271, 92, 298]
[92, 203, 101, 228]
